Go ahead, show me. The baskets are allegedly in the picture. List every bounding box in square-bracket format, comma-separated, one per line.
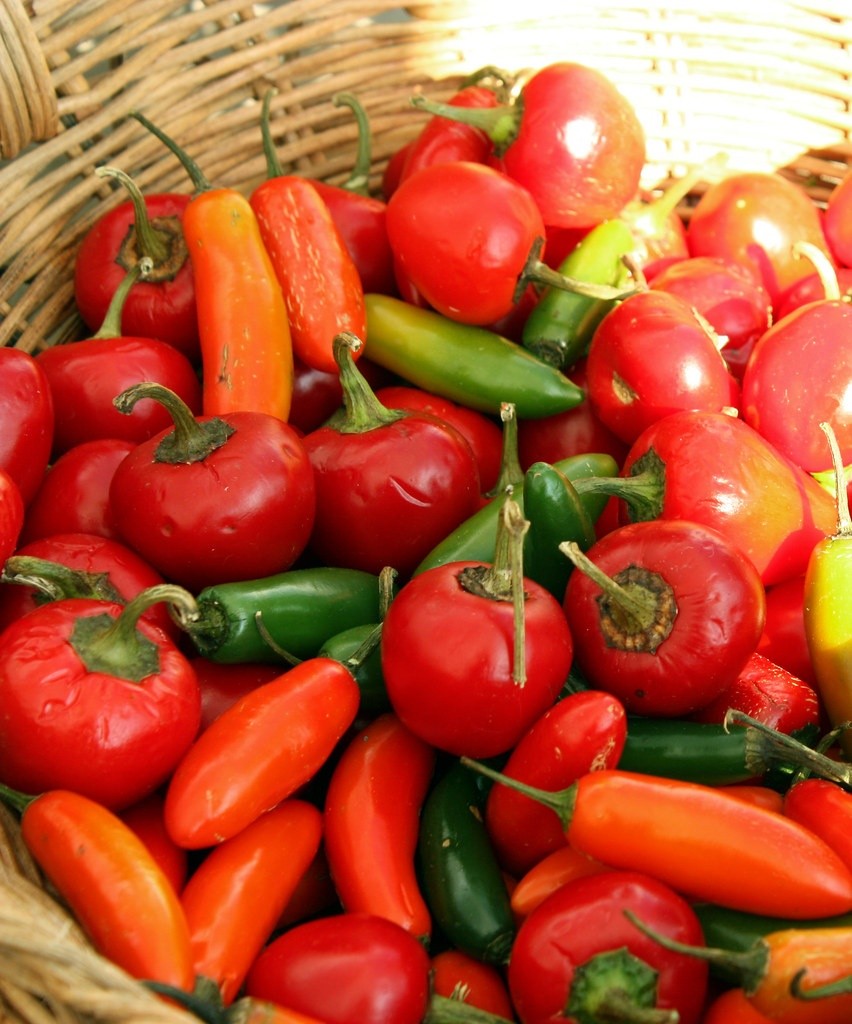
[0, 0, 852, 1024]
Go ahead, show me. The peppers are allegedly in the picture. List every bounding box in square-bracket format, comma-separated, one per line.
[0, 63, 852, 1024]
